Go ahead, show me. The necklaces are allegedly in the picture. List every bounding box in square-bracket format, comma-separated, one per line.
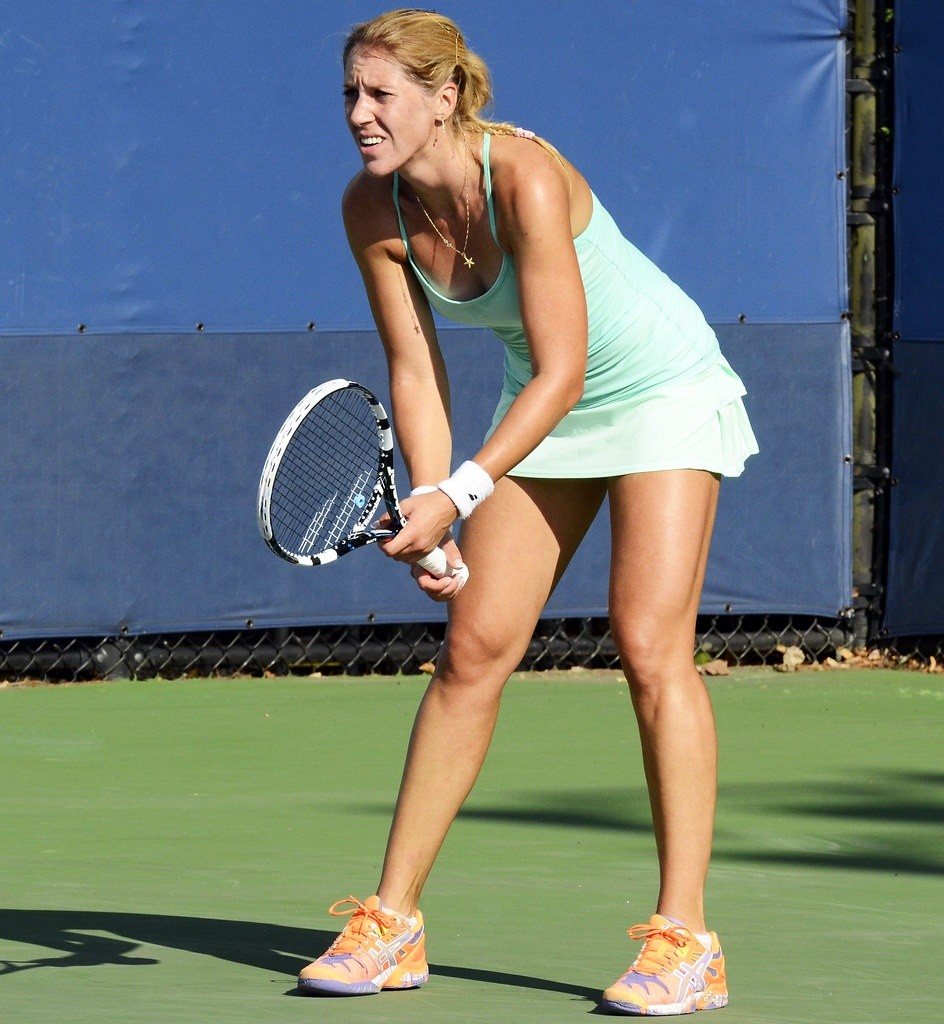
[413, 135, 475, 269]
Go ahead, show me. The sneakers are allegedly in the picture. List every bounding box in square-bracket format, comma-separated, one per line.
[602, 913, 730, 1016]
[298, 894, 429, 996]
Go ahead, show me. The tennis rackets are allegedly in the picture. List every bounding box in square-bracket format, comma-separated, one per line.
[255, 376, 469, 597]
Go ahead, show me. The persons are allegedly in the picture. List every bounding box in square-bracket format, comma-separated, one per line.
[296, 9, 760, 1016]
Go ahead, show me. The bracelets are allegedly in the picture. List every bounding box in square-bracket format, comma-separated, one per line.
[410, 460, 494, 520]
